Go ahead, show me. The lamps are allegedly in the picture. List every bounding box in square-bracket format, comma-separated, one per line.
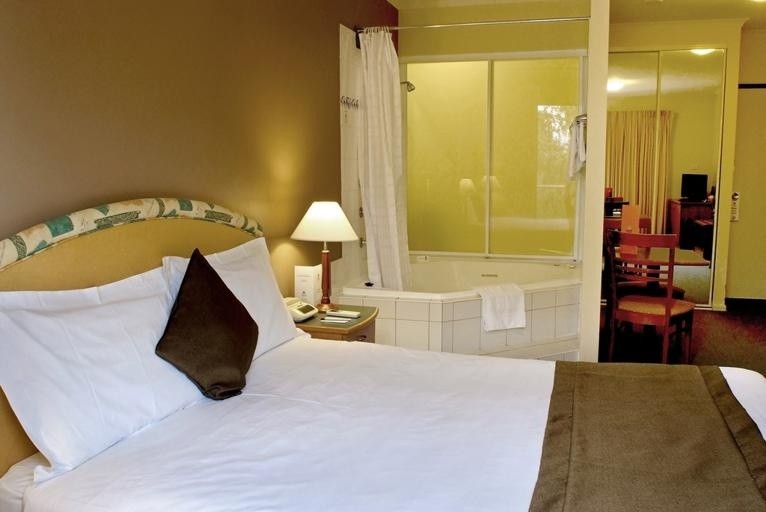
[287, 198, 361, 317]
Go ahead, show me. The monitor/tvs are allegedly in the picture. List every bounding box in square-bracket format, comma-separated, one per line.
[680, 174, 708, 201]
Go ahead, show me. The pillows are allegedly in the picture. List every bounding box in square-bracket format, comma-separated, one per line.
[159, 234, 314, 376]
[1, 262, 208, 482]
[155, 246, 259, 402]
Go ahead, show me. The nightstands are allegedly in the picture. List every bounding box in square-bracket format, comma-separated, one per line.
[294, 298, 380, 345]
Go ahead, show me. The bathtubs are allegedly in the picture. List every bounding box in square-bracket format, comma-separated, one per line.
[344, 250, 582, 303]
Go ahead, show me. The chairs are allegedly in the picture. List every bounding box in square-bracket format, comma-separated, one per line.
[604, 188, 696, 364]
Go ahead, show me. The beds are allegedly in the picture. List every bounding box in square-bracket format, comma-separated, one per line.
[1, 191, 766, 512]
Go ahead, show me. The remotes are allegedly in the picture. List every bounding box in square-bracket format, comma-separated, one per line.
[326, 308, 361, 318]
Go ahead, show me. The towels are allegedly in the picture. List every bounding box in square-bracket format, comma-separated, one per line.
[567, 117, 588, 181]
[468, 281, 528, 333]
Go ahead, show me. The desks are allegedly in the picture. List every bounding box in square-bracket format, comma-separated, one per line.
[669, 197, 712, 250]
[695, 217, 712, 260]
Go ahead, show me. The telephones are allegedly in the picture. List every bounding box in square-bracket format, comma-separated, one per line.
[283, 296, 318, 322]
